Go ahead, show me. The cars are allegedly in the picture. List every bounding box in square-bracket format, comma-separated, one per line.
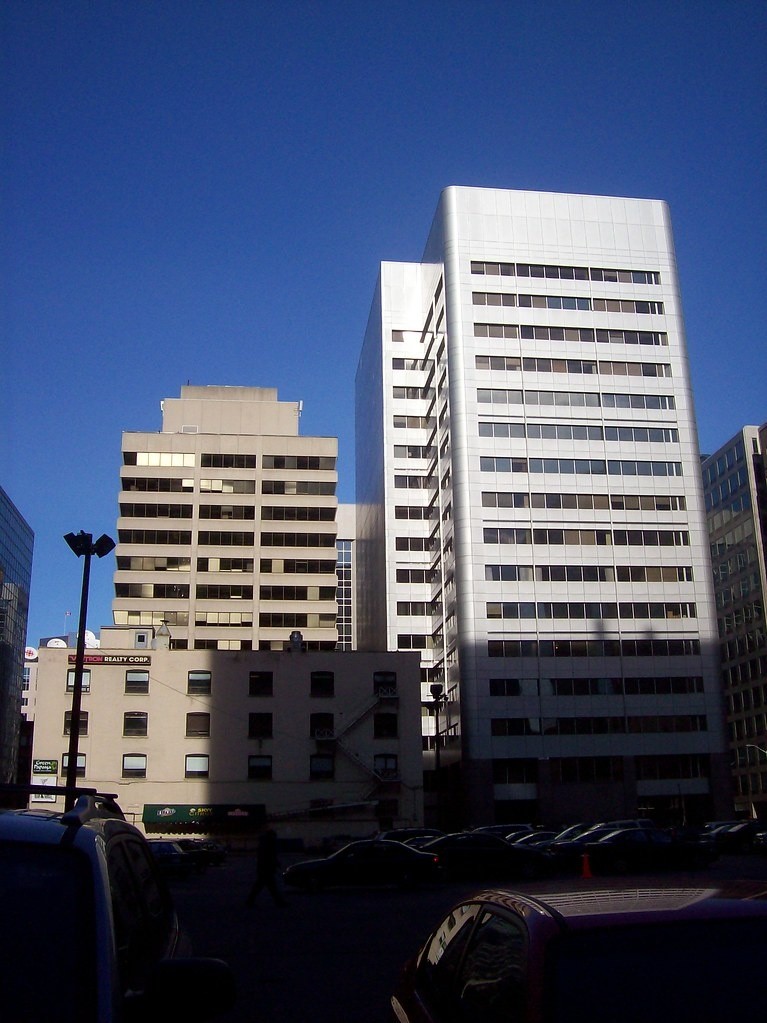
[323, 816, 767, 877]
[147, 838, 230, 870]
[274, 838, 452, 890]
[388, 878, 767, 1023]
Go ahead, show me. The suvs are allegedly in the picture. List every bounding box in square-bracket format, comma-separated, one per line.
[0, 793, 239, 1023]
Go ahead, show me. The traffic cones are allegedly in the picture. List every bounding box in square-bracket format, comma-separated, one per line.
[579, 852, 594, 880]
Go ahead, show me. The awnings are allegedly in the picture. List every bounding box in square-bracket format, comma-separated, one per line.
[143, 804, 266, 825]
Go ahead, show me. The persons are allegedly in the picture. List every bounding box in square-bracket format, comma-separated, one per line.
[244, 829, 291, 911]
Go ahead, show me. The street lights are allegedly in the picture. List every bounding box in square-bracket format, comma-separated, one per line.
[63, 531, 118, 812]
[425, 684, 448, 771]
